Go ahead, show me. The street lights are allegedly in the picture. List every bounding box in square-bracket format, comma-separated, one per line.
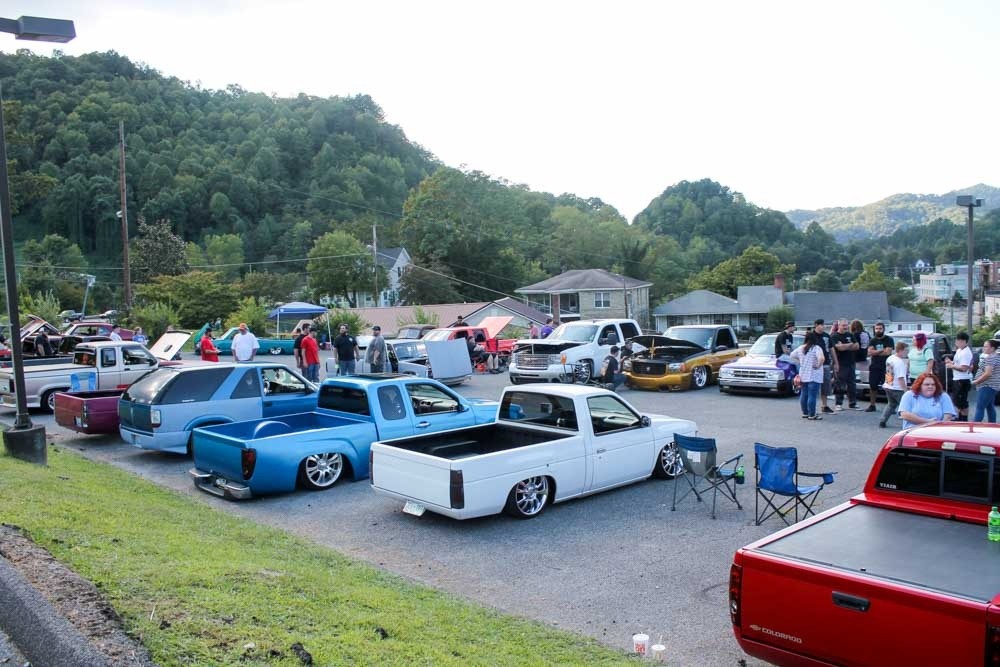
[956, 194, 987, 350]
[0, 15, 84, 468]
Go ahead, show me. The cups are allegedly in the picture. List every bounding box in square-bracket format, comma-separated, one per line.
[633, 634, 649, 657]
[651, 645, 666, 667]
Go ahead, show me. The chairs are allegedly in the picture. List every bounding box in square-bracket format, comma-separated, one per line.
[671, 432, 743, 519]
[556, 417, 602, 433]
[754, 442, 838, 526]
[397, 348, 409, 359]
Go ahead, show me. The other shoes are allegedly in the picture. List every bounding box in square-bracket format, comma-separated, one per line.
[879, 421, 886, 428]
[803, 404, 877, 420]
[950, 413, 968, 421]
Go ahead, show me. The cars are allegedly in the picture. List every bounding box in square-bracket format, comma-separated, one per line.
[393, 324, 438, 342]
[192, 324, 298, 357]
[854, 329, 977, 400]
[716, 330, 808, 398]
[114, 359, 325, 457]
[325, 337, 474, 391]
[622, 324, 750, 393]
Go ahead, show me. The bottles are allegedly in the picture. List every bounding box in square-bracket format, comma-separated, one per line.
[738, 466, 744, 484]
[987, 506, 1000, 541]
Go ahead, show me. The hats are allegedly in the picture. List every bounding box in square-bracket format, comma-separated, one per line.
[239, 323, 248, 330]
[112, 324, 121, 329]
[39, 327, 48, 332]
[784, 321, 795, 330]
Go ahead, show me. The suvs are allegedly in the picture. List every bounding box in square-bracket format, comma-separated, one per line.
[506, 316, 647, 386]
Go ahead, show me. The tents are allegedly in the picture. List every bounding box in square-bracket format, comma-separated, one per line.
[268, 302, 332, 351]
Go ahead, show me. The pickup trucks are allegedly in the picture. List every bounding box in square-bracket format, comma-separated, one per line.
[367, 380, 700, 519]
[189, 373, 528, 501]
[0, 307, 196, 415]
[413, 325, 523, 373]
[728, 420, 1000, 666]
[51, 360, 188, 435]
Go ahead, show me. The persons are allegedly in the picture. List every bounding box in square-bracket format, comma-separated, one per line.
[230, 323, 260, 362]
[453, 316, 504, 374]
[201, 328, 220, 362]
[600, 329, 634, 393]
[208, 320, 216, 339]
[364, 326, 386, 373]
[333, 324, 360, 377]
[294, 323, 321, 383]
[775, 318, 1000, 430]
[528, 318, 553, 339]
[34, 327, 52, 356]
[109, 324, 182, 361]
